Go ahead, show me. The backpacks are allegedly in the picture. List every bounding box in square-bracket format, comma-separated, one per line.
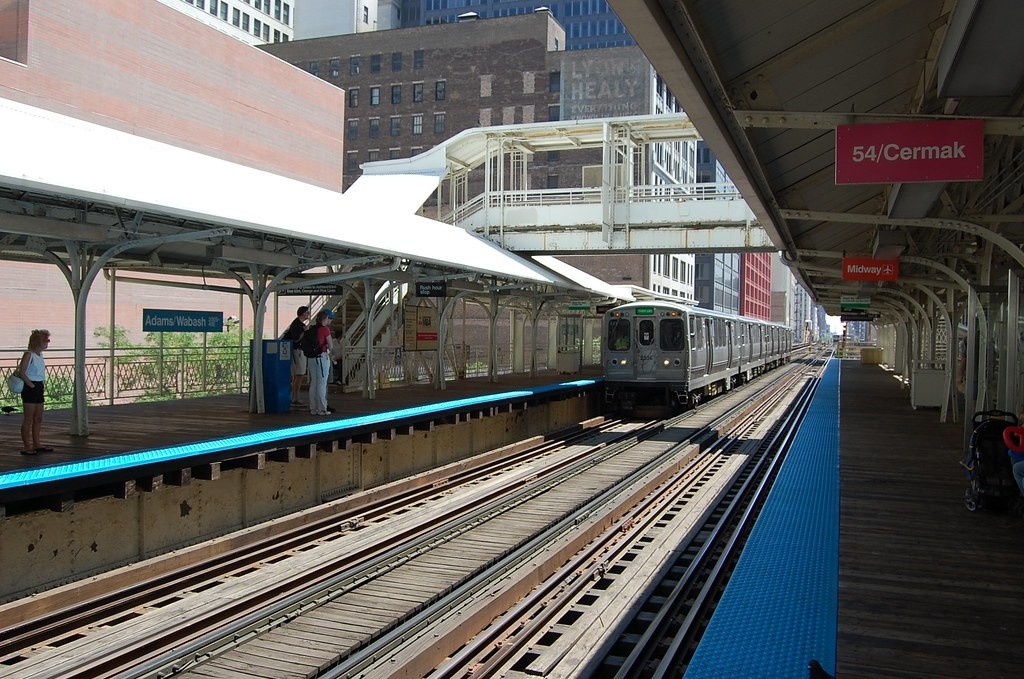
[302, 324, 327, 358]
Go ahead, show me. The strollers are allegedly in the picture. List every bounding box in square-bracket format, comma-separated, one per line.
[961, 409, 1020, 513]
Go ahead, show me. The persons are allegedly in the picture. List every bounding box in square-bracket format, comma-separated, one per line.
[20, 328, 54, 454]
[282, 306, 337, 416]
[614, 332, 630, 350]
[958, 316, 981, 410]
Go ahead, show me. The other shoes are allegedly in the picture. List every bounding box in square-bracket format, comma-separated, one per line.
[336, 380, 342, 385]
[311, 409, 319, 415]
[290, 399, 308, 406]
[317, 409, 332, 416]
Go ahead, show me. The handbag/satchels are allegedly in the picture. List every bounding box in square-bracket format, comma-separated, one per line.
[282, 319, 304, 350]
[7, 352, 32, 394]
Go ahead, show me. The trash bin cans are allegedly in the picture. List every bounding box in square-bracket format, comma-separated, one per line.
[250, 340, 293, 415]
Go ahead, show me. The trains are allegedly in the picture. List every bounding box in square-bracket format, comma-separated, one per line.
[600, 297, 796, 411]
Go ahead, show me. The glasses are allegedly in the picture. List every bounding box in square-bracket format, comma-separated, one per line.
[43, 339, 50, 343]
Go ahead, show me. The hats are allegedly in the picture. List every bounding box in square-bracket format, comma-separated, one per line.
[324, 308, 335, 319]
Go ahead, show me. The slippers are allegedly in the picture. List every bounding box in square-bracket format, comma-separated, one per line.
[34, 445, 53, 451]
[20, 448, 39, 456]
